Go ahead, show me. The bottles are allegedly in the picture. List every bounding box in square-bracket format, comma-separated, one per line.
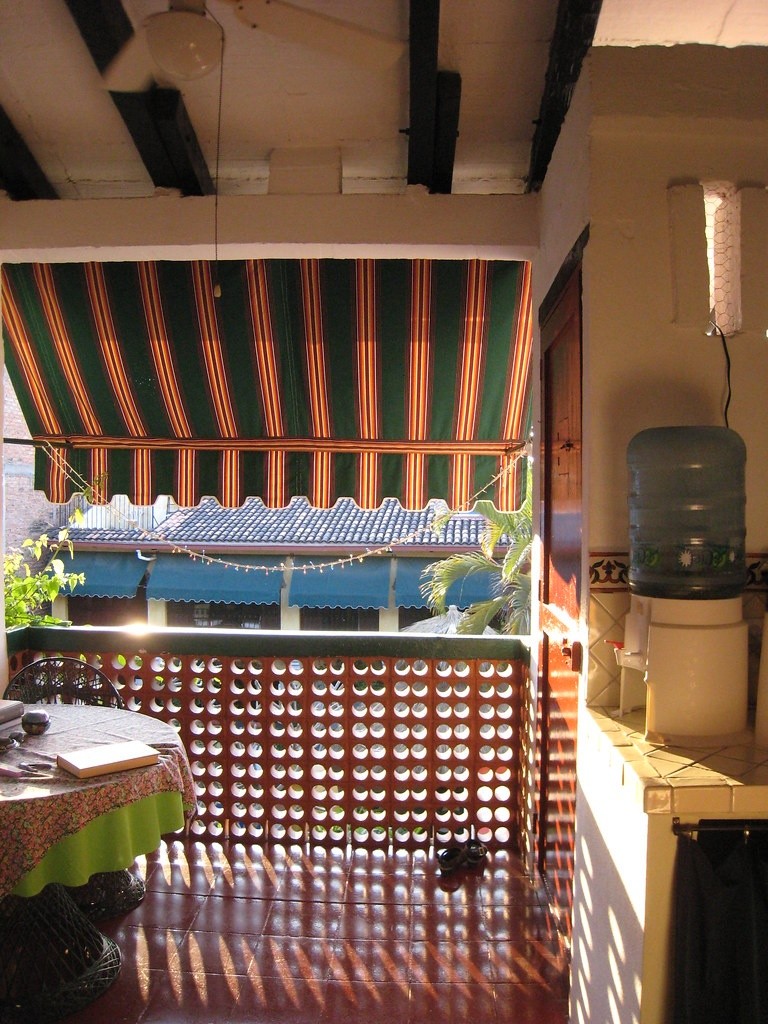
[620, 425, 748, 600]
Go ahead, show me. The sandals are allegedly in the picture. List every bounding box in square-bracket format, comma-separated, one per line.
[467, 839, 487, 868]
[439, 847, 468, 876]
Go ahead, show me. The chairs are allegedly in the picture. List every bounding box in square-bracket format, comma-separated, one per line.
[3, 656, 125, 710]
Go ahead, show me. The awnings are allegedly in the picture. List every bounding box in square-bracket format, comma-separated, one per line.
[42, 550, 496, 611]
[2, 260, 533, 514]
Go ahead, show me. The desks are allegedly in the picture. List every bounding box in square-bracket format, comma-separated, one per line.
[568, 704, 768, 1024]
[0, 703, 197, 1024]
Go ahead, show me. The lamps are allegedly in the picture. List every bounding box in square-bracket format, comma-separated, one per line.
[141, 0, 226, 82]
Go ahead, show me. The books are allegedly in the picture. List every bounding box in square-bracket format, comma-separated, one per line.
[0, 699, 24, 725]
[57, 739, 159, 779]
